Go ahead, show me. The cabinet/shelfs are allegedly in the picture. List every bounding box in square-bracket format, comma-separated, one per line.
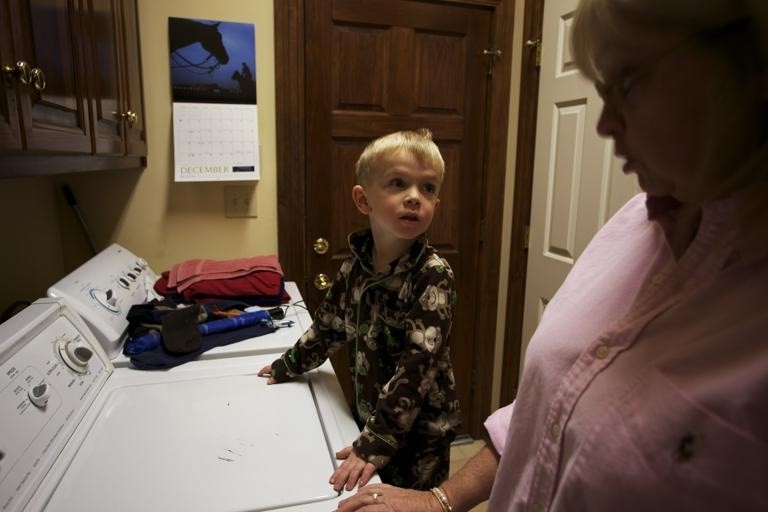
[0, 1, 94, 180]
[82, 2, 151, 173]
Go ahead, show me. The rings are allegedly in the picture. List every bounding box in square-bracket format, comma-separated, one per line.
[371, 493, 378, 505]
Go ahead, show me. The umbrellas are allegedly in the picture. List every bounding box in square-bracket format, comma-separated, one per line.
[126, 307, 284, 355]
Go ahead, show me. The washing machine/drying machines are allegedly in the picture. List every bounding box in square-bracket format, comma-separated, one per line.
[46, 241, 314, 368]
[0, 296, 384, 512]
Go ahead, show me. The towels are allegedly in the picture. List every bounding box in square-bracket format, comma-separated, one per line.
[166, 254, 290, 296]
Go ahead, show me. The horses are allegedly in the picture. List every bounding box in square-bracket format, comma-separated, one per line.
[231, 70, 256, 98]
[169, 17, 231, 66]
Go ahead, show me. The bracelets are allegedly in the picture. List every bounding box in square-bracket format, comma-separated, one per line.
[429, 486, 452, 512]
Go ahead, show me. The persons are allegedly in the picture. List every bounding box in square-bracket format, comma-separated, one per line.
[331, 0, 767, 512]
[241, 61, 252, 81]
[258, 127, 460, 494]
